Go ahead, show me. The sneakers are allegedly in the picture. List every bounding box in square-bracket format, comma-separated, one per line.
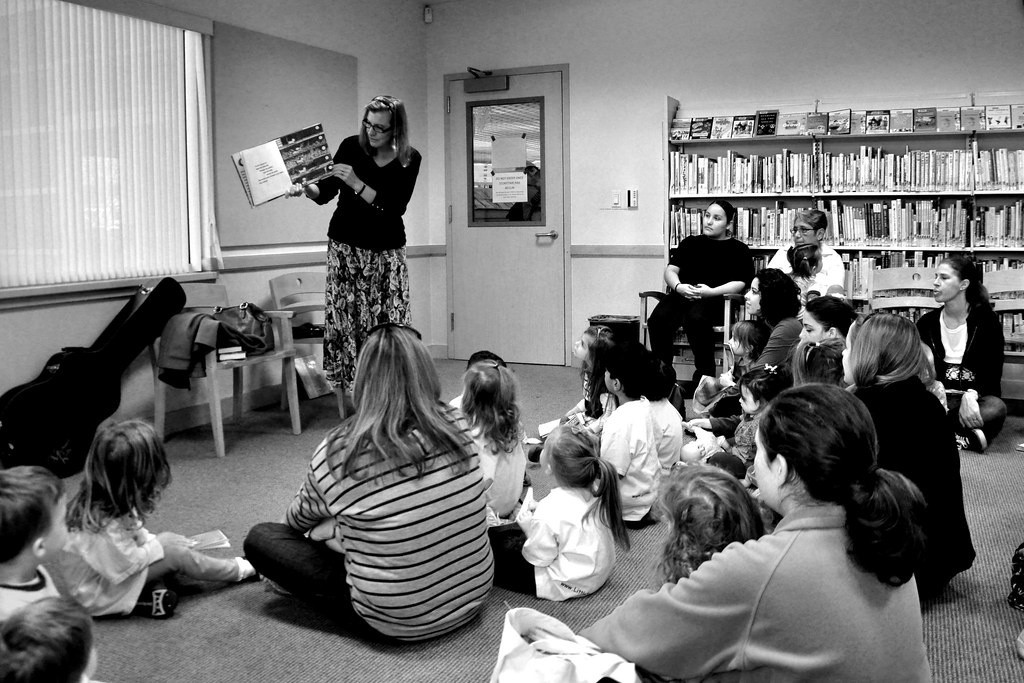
[954, 428, 987, 451]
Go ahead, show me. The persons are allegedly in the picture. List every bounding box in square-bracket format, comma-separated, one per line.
[447, 350, 527, 520]
[645, 201, 977, 605]
[529, 170, 540, 221]
[528, 326, 683, 527]
[56, 418, 264, 618]
[647, 200, 755, 399]
[243, 323, 494, 646]
[285, 91, 423, 392]
[576, 383, 932, 683]
[487, 425, 630, 603]
[0, 464, 98, 683]
[914, 254, 1007, 454]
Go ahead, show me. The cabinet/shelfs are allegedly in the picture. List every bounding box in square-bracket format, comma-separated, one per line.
[664, 93, 1024, 400]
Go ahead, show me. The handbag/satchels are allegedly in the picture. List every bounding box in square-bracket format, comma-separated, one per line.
[1008, 542, 1024, 612]
[489, 607, 643, 683]
[212, 302, 275, 351]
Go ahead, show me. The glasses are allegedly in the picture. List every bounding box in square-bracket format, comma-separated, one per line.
[362, 118, 393, 133]
[364, 322, 421, 340]
[790, 227, 819, 234]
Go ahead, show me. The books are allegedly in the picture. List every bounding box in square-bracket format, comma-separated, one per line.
[668, 140, 1024, 248]
[752, 251, 1024, 352]
[671, 104, 1024, 140]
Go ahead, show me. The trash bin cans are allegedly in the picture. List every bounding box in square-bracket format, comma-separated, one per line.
[588, 315, 641, 342]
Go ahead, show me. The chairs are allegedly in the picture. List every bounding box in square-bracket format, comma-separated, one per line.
[269, 270, 349, 422]
[140, 283, 301, 460]
[635, 269, 1024, 400]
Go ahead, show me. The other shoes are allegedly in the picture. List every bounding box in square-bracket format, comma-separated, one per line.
[523, 475, 532, 486]
[133, 590, 179, 619]
[527, 445, 544, 462]
[242, 554, 265, 581]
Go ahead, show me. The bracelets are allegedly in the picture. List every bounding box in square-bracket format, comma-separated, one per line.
[354, 184, 367, 195]
[675, 283, 681, 291]
